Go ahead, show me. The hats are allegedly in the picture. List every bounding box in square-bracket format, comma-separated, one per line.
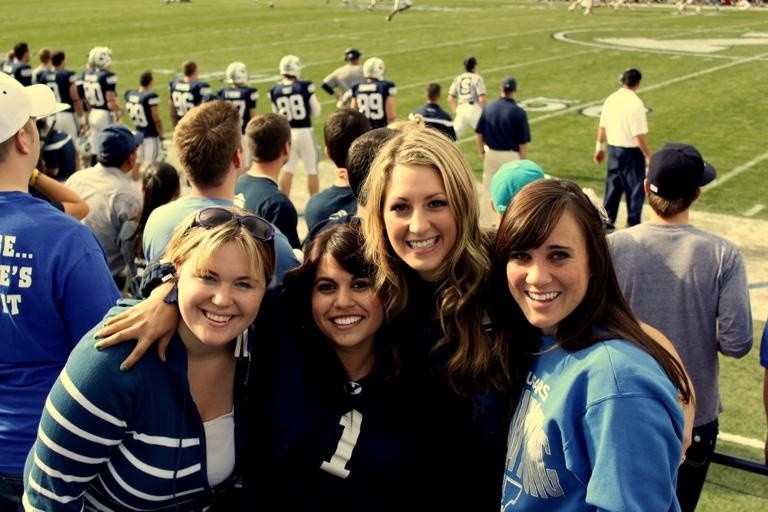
[0, 70, 70, 146]
[648, 143, 717, 197]
[490, 161, 545, 213]
[96, 125, 144, 158]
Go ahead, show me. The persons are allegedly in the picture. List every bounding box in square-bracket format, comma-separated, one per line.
[253, 220, 408, 512]
[594, 68, 656, 232]
[490, 179, 692, 512]
[604, 144, 754, 512]
[0, 71, 122, 512]
[1, 41, 546, 294]
[23, 205, 277, 512]
[100, 128, 696, 512]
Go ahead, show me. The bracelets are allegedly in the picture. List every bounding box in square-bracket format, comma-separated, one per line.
[597, 142, 606, 151]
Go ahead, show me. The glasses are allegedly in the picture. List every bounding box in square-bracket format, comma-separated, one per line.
[184, 206, 276, 260]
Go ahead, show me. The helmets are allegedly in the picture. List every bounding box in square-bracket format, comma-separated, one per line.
[226, 62, 246, 84]
[279, 55, 302, 79]
[89, 46, 112, 72]
[363, 57, 385, 81]
